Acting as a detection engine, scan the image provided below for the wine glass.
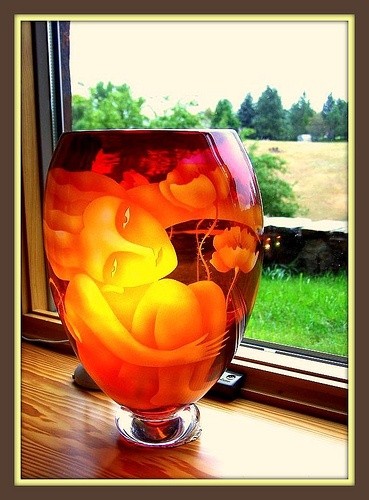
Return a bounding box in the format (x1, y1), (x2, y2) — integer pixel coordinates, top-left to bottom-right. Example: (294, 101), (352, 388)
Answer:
(42, 128), (263, 449)
